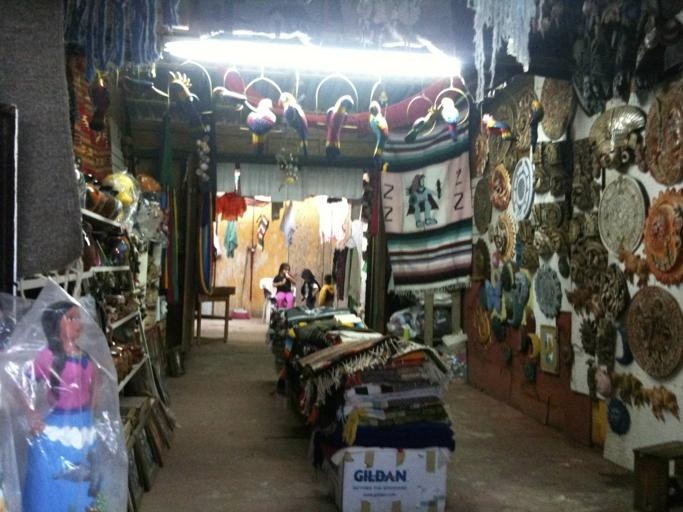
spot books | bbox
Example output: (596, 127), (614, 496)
(118, 391), (179, 511)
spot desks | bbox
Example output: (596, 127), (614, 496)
(192, 286), (236, 346)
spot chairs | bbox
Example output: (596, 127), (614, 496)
(257, 278), (297, 328)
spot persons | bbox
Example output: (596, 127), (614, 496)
(299, 269), (321, 308)
(5, 302), (103, 511)
(317, 274), (333, 307)
(271, 263), (297, 309)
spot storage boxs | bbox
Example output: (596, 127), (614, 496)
(316, 446), (448, 511)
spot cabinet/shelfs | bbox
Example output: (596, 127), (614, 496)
(81, 208), (156, 408)
